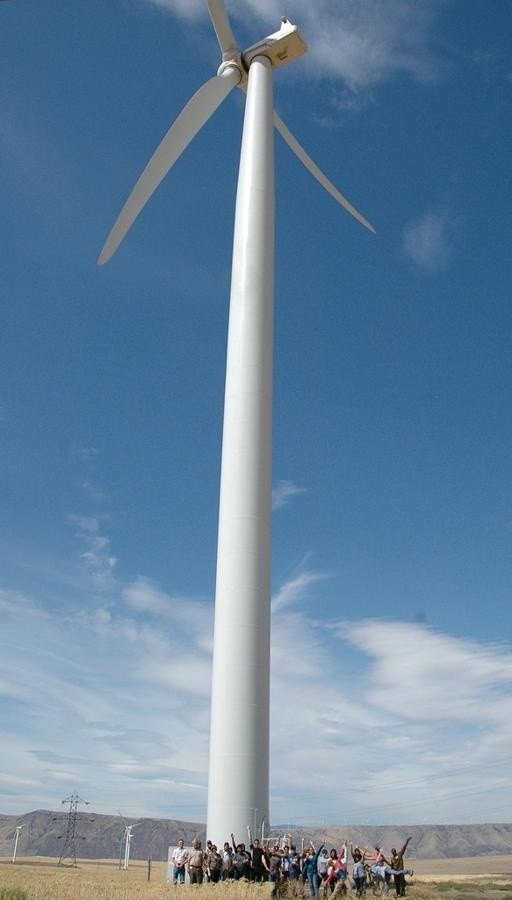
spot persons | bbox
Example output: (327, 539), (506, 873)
(168, 823), (416, 900)
